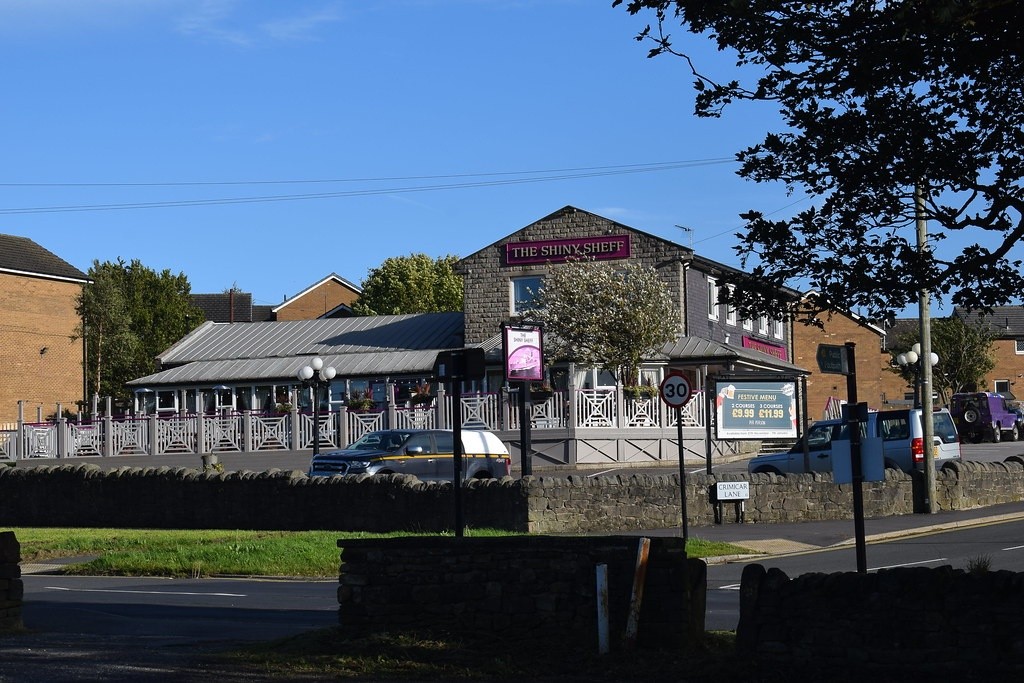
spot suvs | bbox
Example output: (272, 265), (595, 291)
(950, 390), (1024, 443)
(748, 409), (961, 475)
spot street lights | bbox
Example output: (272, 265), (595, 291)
(897, 343), (939, 408)
(298, 358), (337, 455)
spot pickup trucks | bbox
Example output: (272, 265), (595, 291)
(306, 431), (513, 480)
